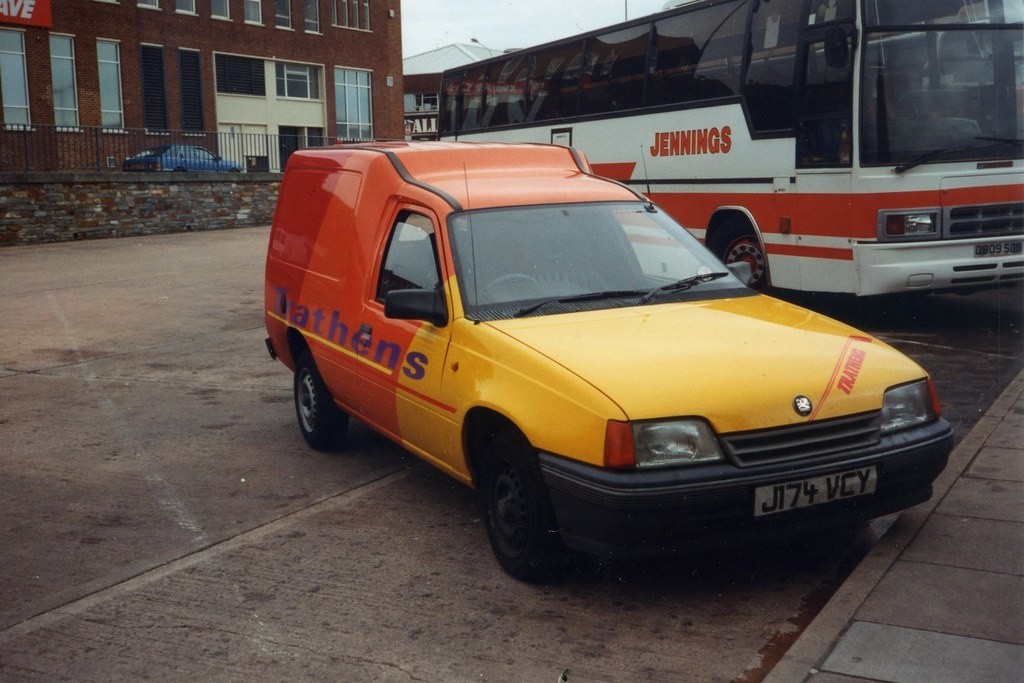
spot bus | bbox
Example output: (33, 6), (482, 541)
(435, 1), (1024, 308)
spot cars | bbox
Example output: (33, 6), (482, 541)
(121, 144), (244, 173)
(263, 142), (956, 587)
